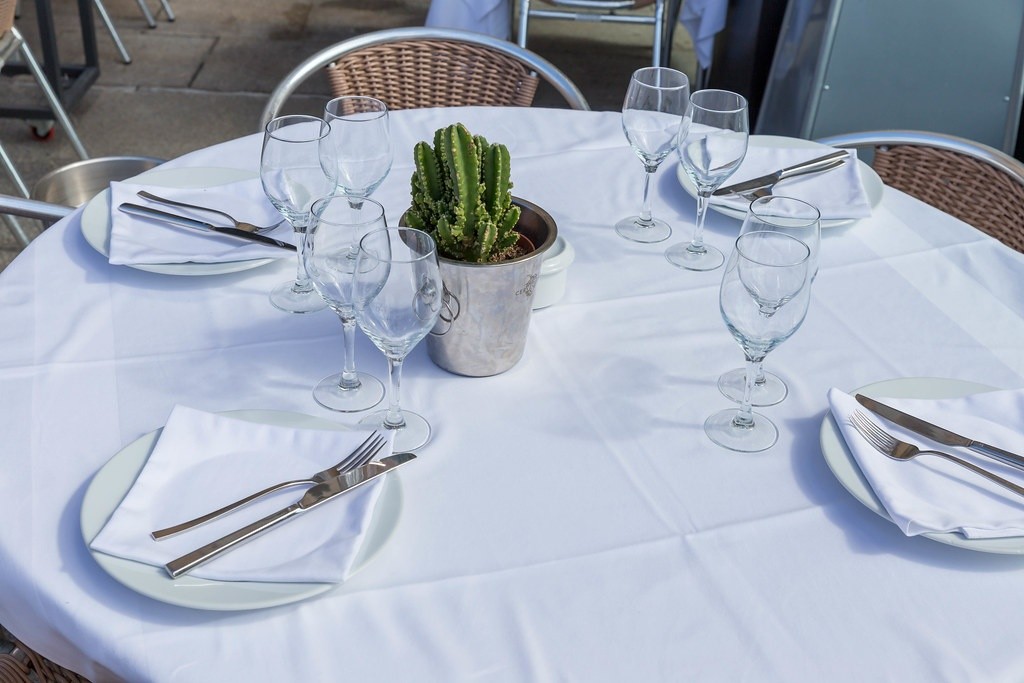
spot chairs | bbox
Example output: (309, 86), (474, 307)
(0, 0), (176, 248)
(260, 27), (590, 131)
(812, 129), (1024, 256)
(519, 0), (681, 112)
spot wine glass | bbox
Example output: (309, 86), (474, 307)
(304, 187), (387, 413)
(613, 67), (693, 242)
(349, 226), (442, 456)
(317, 95), (395, 274)
(703, 227), (814, 453)
(259, 117), (344, 313)
(664, 88), (750, 270)
(717, 197), (821, 405)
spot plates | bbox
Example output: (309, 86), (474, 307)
(79, 163), (298, 277)
(818, 374), (1024, 556)
(676, 134), (885, 229)
(82, 406), (405, 611)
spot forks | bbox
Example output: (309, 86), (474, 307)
(150, 423), (387, 545)
(728, 160), (847, 204)
(137, 188), (287, 235)
(846, 408), (1024, 505)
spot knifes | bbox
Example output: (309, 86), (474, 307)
(118, 202), (298, 255)
(852, 392), (1024, 473)
(164, 451), (419, 580)
(699, 150), (851, 198)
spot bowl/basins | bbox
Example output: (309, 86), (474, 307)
(27, 157), (168, 230)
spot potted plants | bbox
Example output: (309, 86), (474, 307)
(398, 123), (558, 380)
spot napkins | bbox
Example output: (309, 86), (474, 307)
(108, 175), (299, 266)
(827, 385), (1024, 541)
(91, 401), (399, 584)
(704, 133), (871, 221)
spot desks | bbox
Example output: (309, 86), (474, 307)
(0, 108), (1024, 683)
